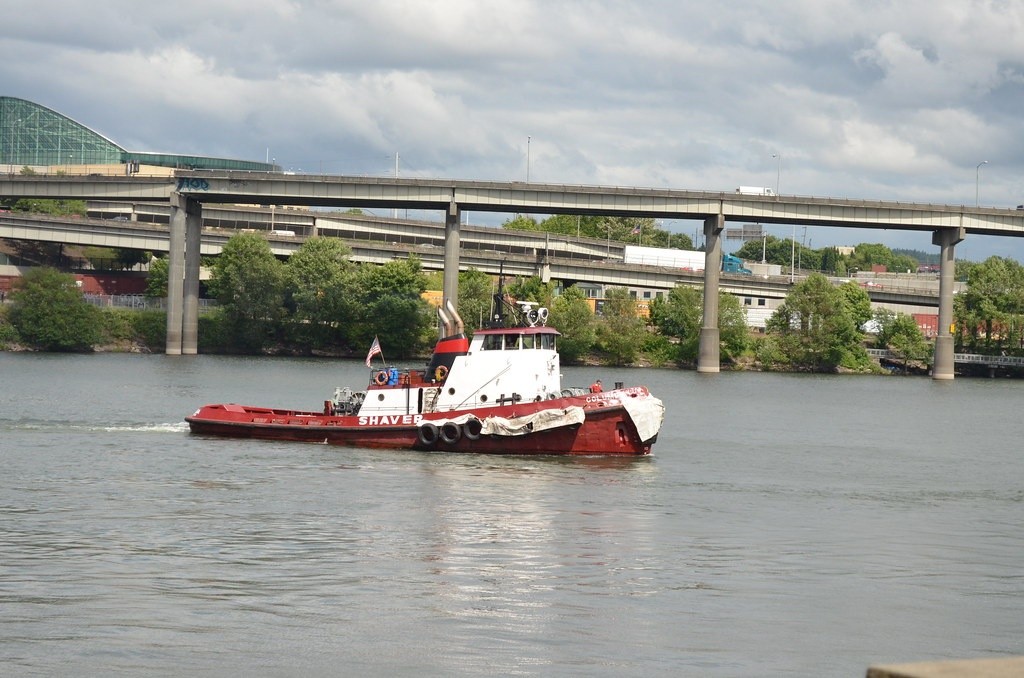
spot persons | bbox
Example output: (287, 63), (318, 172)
(589, 380), (603, 393)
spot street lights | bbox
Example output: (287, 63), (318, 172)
(976, 161), (988, 206)
(773, 154), (782, 195)
(527, 136), (531, 183)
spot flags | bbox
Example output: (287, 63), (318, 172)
(366, 334), (381, 368)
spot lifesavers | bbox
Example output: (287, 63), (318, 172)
(434, 364), (449, 380)
(374, 371), (390, 385)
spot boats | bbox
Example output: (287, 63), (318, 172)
(184, 257), (666, 455)
